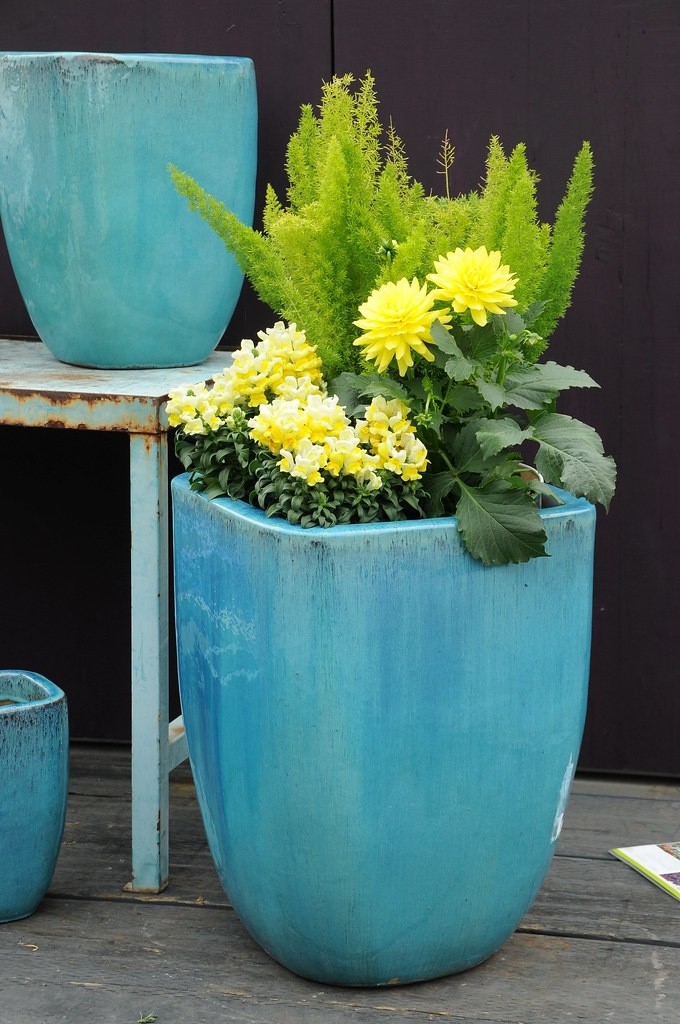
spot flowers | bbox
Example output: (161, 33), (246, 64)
(163, 65), (615, 568)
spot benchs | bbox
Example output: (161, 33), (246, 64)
(0, 339), (245, 890)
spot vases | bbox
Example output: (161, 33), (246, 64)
(0, 52), (257, 370)
(0, 670), (70, 924)
(172, 473), (598, 991)
